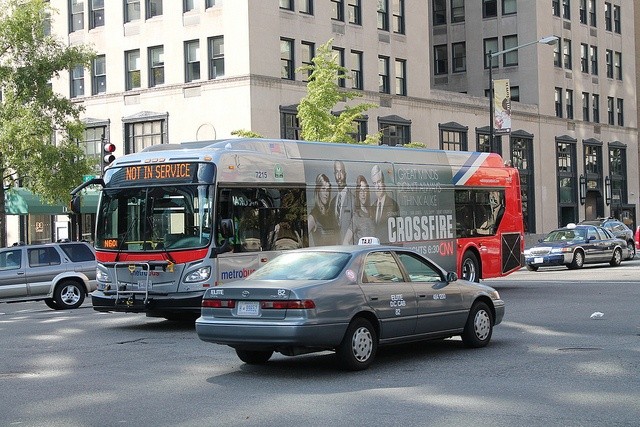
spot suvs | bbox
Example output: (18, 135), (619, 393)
(0, 241), (97, 311)
(576, 217), (636, 261)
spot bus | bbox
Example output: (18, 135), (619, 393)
(91, 137), (526, 318)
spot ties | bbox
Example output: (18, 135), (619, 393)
(337, 194), (342, 222)
(374, 199), (384, 227)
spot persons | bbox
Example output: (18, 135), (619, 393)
(369, 165), (400, 243)
(342, 176), (377, 245)
(306, 172), (338, 245)
(328, 159), (356, 245)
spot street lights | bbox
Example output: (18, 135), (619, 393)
(486, 34), (559, 152)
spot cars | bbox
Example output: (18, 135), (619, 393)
(195, 237), (506, 373)
(525, 222), (629, 272)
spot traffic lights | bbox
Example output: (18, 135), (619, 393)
(101, 142), (116, 175)
